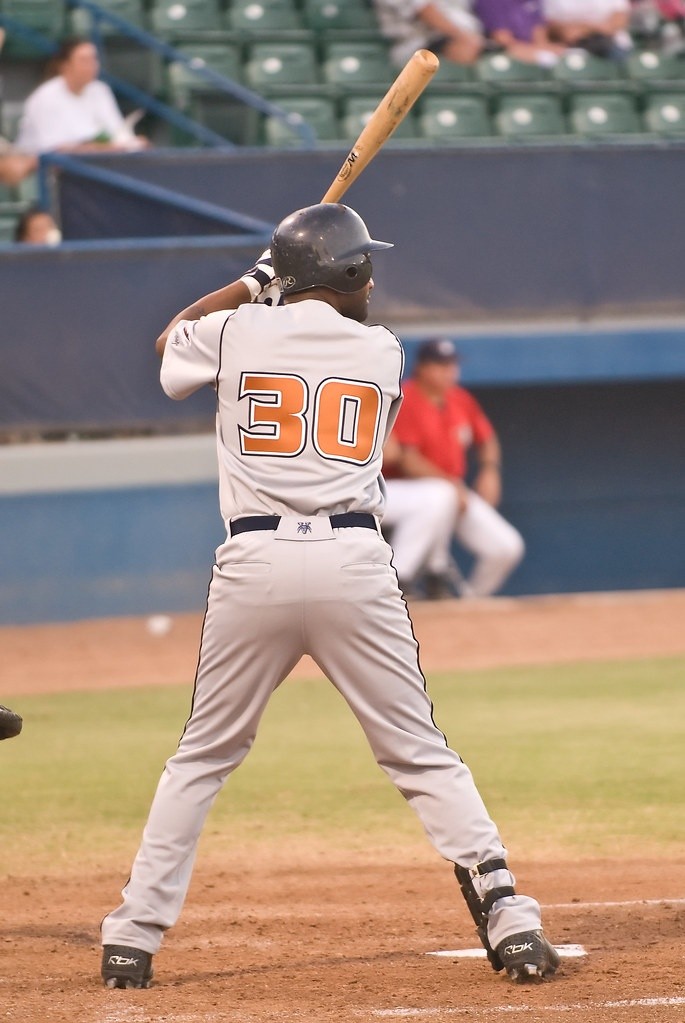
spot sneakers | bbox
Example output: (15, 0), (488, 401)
(101, 944), (154, 989)
(496, 929), (561, 983)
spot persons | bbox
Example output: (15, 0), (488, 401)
(13, 212), (62, 246)
(99, 204), (561, 988)
(373, 0), (685, 68)
(381, 336), (526, 601)
(15, 37), (145, 203)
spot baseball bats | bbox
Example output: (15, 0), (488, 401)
(320, 48), (440, 202)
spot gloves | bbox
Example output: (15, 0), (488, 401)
(240, 249), (276, 303)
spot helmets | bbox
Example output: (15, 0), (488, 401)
(271, 202), (394, 296)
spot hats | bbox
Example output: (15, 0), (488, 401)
(421, 340), (457, 364)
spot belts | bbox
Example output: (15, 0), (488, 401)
(230, 513), (377, 536)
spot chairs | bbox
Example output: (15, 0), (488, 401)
(0, 0), (685, 241)
